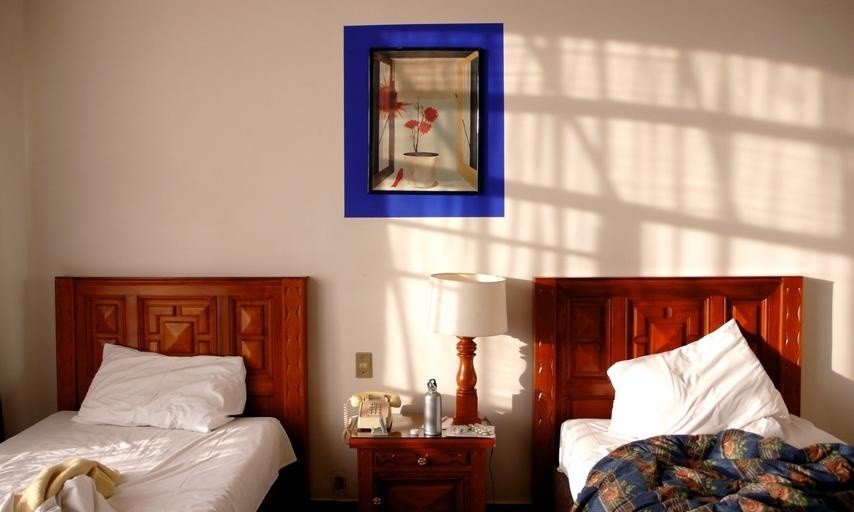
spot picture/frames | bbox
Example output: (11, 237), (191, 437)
(340, 22), (507, 220)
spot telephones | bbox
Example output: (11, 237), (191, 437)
(351, 391), (401, 432)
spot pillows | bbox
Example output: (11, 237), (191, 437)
(68, 341), (250, 434)
(603, 316), (792, 454)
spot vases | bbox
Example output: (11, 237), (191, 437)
(401, 154), (439, 190)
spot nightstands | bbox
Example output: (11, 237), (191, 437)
(347, 413), (496, 511)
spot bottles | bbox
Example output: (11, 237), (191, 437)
(424, 379), (441, 435)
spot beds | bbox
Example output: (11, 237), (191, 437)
(527, 273), (854, 511)
(0, 276), (313, 512)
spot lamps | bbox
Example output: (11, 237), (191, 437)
(428, 272), (510, 426)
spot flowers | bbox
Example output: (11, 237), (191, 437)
(401, 92), (441, 154)
